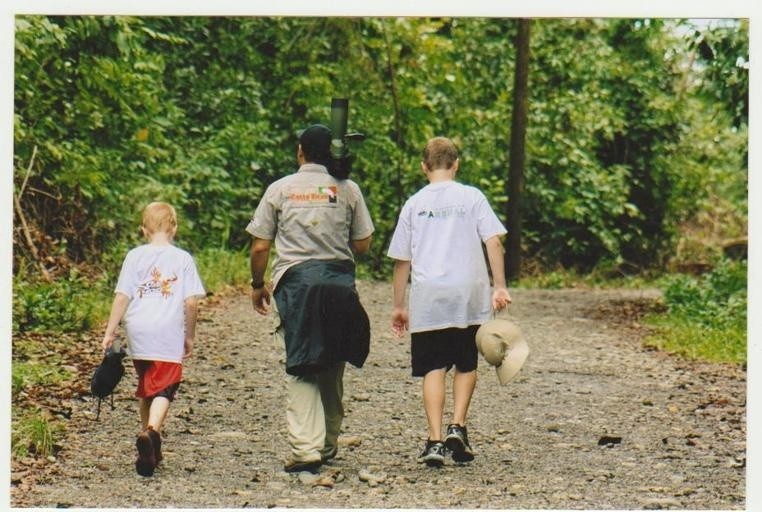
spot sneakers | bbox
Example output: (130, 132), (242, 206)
(135, 426), (162, 477)
(416, 437), (446, 464)
(444, 423), (474, 462)
(284, 457), (321, 474)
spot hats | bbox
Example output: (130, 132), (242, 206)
(295, 124), (333, 149)
(91, 351), (126, 399)
(475, 318), (530, 386)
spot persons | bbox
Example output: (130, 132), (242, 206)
(246, 125), (376, 471)
(101, 201), (206, 476)
(387, 137), (512, 464)
